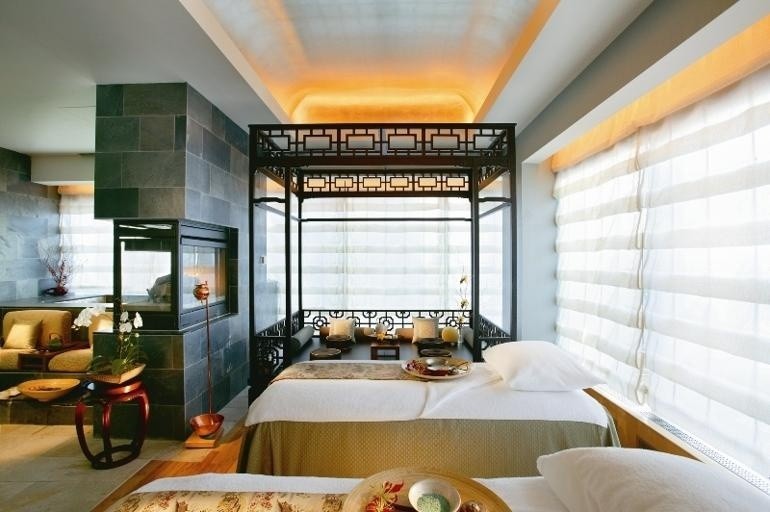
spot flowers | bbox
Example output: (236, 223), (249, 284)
(86, 311), (150, 374)
(456, 275), (471, 326)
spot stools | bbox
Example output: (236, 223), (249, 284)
(309, 347), (342, 359)
(75, 377), (149, 468)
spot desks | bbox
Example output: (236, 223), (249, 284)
(370, 341), (400, 360)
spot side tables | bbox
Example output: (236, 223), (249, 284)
(18, 341), (88, 372)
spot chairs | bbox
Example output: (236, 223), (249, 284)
(48, 312), (113, 373)
(0, 310), (72, 369)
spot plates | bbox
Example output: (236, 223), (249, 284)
(18, 378), (81, 401)
(427, 366), (454, 374)
(342, 465), (513, 512)
(426, 356), (448, 366)
(401, 357), (473, 379)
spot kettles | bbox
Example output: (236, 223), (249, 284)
(47, 332), (64, 351)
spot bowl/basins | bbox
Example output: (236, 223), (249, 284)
(408, 479), (461, 511)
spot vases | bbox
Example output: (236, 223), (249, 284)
(88, 362), (145, 384)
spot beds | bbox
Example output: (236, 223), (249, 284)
(247, 122), (517, 410)
(237, 359), (621, 479)
(102, 471), (566, 512)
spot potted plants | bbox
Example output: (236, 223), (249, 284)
(38, 236), (69, 295)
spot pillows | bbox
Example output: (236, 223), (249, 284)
(536, 447), (769, 512)
(411, 316), (439, 344)
(460, 327), (473, 347)
(290, 325), (315, 353)
(326, 320), (356, 343)
(482, 340), (608, 392)
(320, 326), (371, 336)
(396, 328), (443, 339)
(2, 318), (43, 349)
(441, 326), (458, 343)
